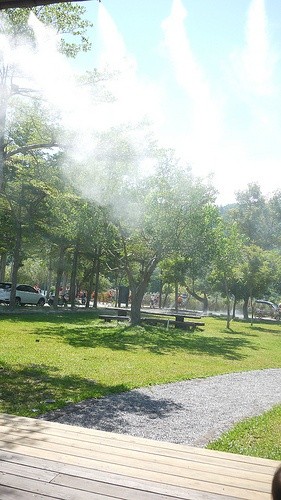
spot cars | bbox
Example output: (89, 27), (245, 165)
(0, 282), (46, 307)
(48, 293), (81, 305)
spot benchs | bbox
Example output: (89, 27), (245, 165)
(98, 315), (205, 330)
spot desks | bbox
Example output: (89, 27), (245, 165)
(104, 307), (201, 328)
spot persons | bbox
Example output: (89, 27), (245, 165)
(151, 295), (155, 304)
(107, 291), (113, 301)
(272, 467), (281, 500)
(82, 292), (86, 304)
(59, 288), (64, 295)
(33, 284), (39, 291)
(178, 295), (183, 308)
